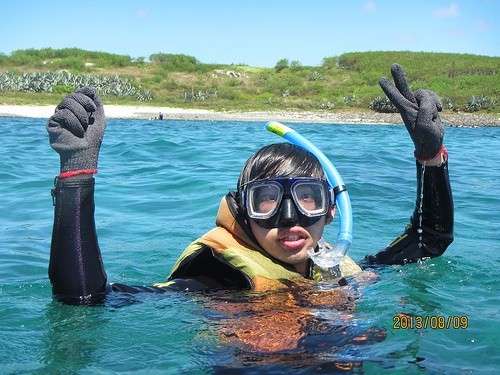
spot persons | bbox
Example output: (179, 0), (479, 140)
(47, 60), (456, 375)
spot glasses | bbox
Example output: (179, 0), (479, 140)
(239, 176), (336, 230)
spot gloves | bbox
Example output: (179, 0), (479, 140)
(44, 84), (105, 180)
(377, 64), (446, 164)
